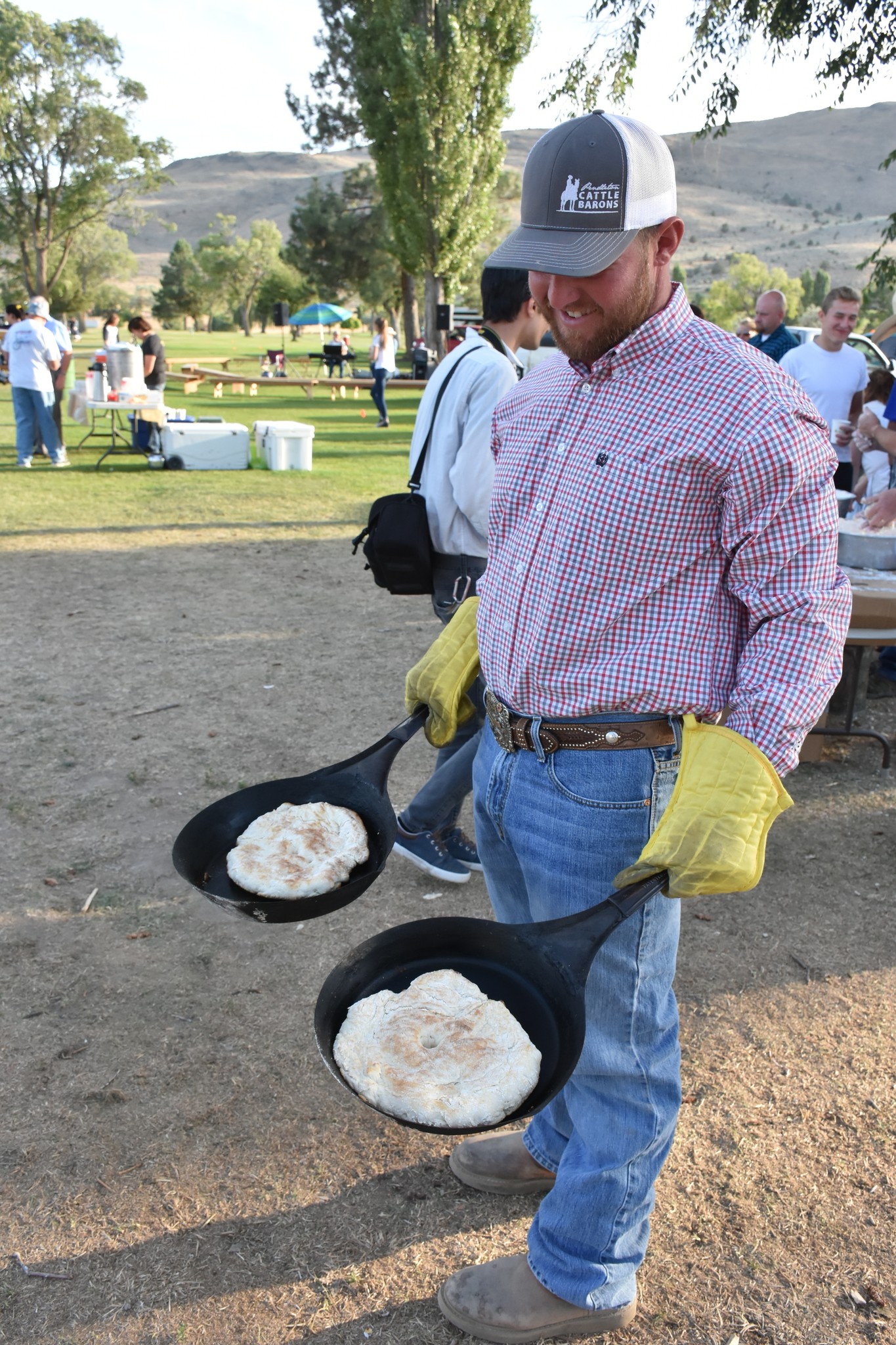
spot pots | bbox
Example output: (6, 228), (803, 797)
(313, 871), (672, 1136)
(170, 702), (429, 924)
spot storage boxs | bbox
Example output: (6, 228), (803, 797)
(253, 421), (315, 472)
(127, 413), (250, 470)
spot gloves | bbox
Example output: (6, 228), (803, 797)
(612, 705), (795, 901)
(405, 595), (484, 748)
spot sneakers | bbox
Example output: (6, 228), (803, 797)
(388, 811), (484, 884)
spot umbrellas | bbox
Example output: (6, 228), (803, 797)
(288, 301), (354, 376)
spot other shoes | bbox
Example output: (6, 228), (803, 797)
(376, 418), (390, 426)
(51, 460), (70, 468)
(17, 462), (32, 468)
(437, 1251), (638, 1344)
(449, 1127), (559, 1195)
(866, 659), (896, 697)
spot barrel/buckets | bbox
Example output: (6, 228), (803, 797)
(105, 347), (145, 391)
(126, 413), (196, 451)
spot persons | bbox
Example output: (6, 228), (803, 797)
(327, 331), (356, 378)
(370, 326), (400, 379)
(367, 316), (396, 428)
(747, 290), (801, 364)
(103, 313), (120, 350)
(128, 316), (167, 454)
(778, 286), (871, 493)
(853, 487), (896, 534)
(0, 302), (69, 468)
(67, 316), (82, 342)
(852, 378), (896, 489)
(4, 296), (73, 460)
(735, 317), (758, 343)
(391, 265), (552, 887)
(404, 112), (852, 1345)
(848, 369), (896, 516)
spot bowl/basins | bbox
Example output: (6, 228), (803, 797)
(148, 457), (165, 469)
(837, 531), (896, 571)
(835, 488), (857, 518)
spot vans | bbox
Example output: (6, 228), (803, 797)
(760, 327), (896, 376)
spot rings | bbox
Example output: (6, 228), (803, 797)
(881, 519), (887, 525)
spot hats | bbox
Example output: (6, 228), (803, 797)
(388, 327), (397, 335)
(24, 304), (50, 322)
(482, 108), (679, 278)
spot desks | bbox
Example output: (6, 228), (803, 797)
(67, 389), (165, 472)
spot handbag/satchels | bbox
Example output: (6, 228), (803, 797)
(351, 492), (437, 596)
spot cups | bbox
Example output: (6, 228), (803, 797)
(829, 418), (851, 444)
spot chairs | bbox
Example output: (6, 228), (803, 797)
(259, 350), (285, 372)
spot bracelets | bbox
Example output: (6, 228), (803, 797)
(871, 424), (881, 438)
(58, 371), (66, 376)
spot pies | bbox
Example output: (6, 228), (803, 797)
(334, 967), (542, 1128)
(226, 802), (369, 898)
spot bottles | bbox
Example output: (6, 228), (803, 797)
(85, 366), (93, 398)
(92, 362), (105, 402)
(95, 349), (107, 364)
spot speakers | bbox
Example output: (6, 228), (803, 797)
(436, 304), (454, 331)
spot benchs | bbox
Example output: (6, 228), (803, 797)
(163, 356), (429, 401)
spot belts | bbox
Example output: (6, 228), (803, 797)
(481, 689), (676, 755)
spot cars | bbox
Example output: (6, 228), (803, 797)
(412, 324), (524, 383)
